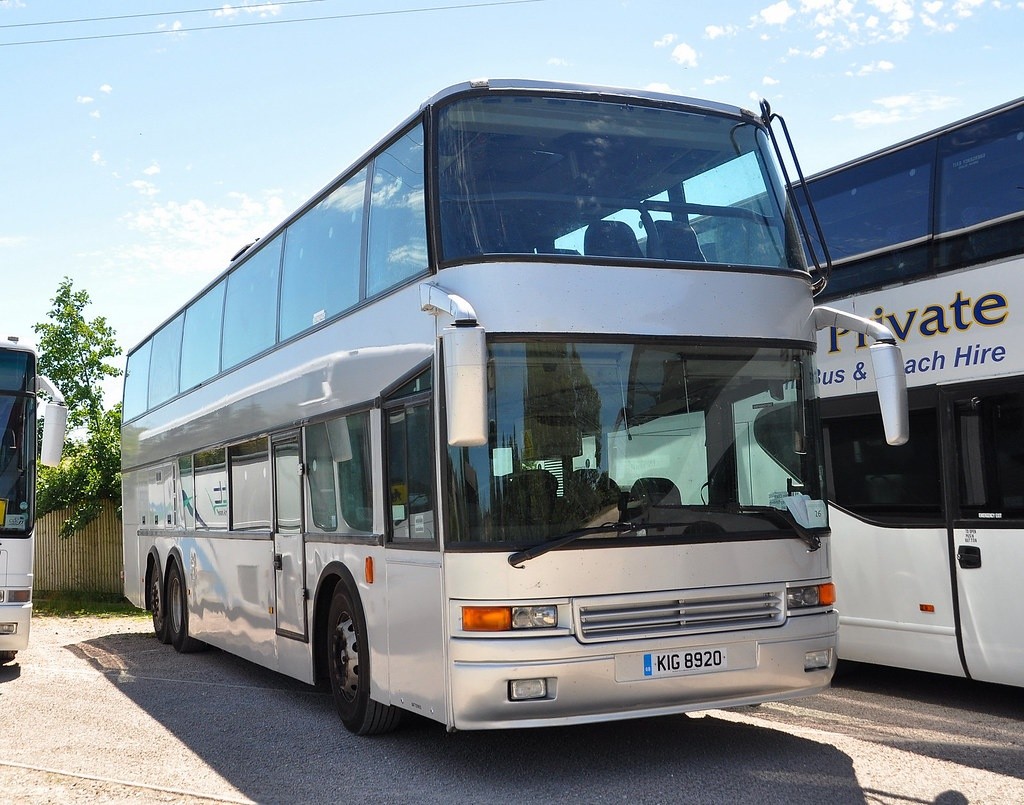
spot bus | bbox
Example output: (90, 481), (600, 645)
(121, 79), (910, 738)
(492, 94), (1024, 693)
(0, 336), (68, 666)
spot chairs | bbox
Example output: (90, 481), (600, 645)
(584, 220), (707, 263)
(484, 469), (682, 540)
